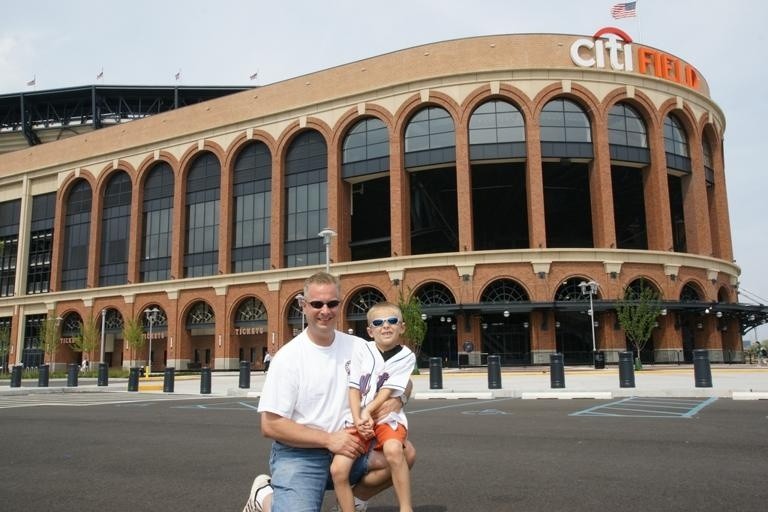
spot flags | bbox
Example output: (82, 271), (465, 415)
(249, 73), (257, 80)
(175, 72), (183, 80)
(612, 1), (637, 20)
(27, 78), (36, 86)
(95, 71), (103, 81)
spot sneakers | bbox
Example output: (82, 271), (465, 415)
(242, 474), (271, 512)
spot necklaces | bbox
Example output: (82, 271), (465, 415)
(308, 328), (335, 346)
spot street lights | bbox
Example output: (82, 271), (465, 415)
(144, 307), (159, 373)
(296, 293), (306, 332)
(101, 308), (106, 363)
(578, 280), (600, 365)
(319, 227), (338, 274)
(749, 315), (759, 343)
(47, 313), (62, 373)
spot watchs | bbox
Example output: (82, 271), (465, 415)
(398, 393), (408, 407)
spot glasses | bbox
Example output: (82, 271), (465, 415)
(369, 317), (399, 328)
(310, 301), (339, 308)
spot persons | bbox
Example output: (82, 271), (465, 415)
(241, 270), (412, 512)
(262, 352), (271, 373)
(754, 341), (768, 368)
(329, 301), (412, 512)
(81, 357), (87, 374)
(85, 358), (89, 373)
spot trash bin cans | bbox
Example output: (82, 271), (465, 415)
(595, 351), (604, 369)
